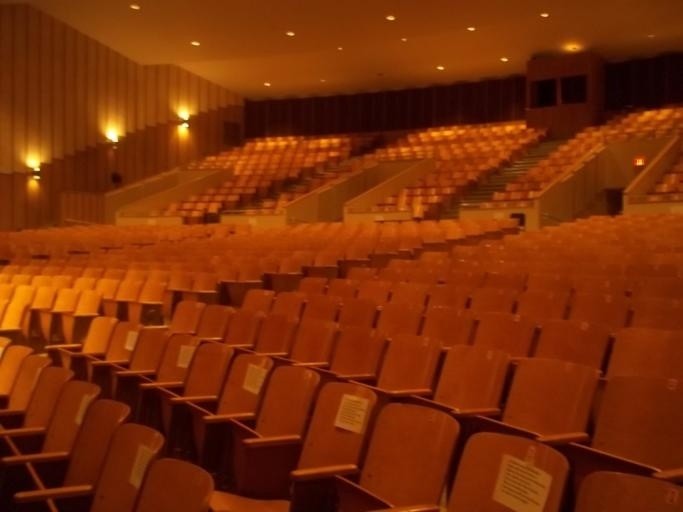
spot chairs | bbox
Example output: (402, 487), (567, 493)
(0, 102), (681, 512)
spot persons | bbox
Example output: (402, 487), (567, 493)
(110, 173), (123, 189)
